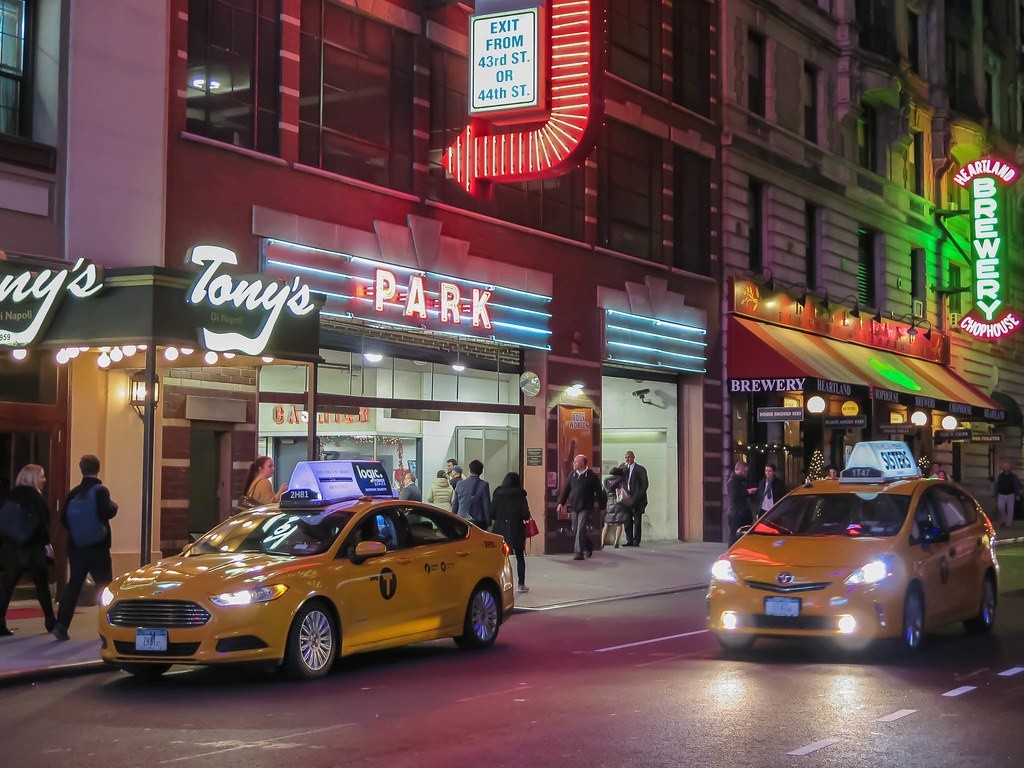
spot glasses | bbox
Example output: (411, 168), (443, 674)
(403, 477), (410, 480)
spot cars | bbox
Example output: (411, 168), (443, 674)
(96, 458), (516, 683)
(706, 441), (1000, 660)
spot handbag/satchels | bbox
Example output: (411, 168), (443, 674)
(0, 501), (41, 542)
(615, 482), (633, 507)
(464, 514), (483, 528)
(522, 516), (539, 538)
(234, 478), (266, 515)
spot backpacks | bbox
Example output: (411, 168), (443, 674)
(67, 484), (108, 546)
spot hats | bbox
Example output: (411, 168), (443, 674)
(452, 466), (462, 473)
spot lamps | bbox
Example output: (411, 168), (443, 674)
(747, 267), (774, 292)
(891, 313), (918, 335)
(933, 416), (958, 447)
(806, 395), (826, 416)
(841, 295), (860, 318)
(917, 320), (932, 341)
(788, 283), (808, 309)
(451, 363), (468, 371)
(809, 286), (829, 311)
(363, 353), (382, 362)
(911, 411), (927, 441)
(860, 303), (882, 325)
(837, 401), (859, 434)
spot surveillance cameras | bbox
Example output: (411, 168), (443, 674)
(632, 389), (650, 397)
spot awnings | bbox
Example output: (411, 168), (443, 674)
(726, 315), (1006, 422)
(990, 392), (1023, 426)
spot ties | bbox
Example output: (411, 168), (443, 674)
(625, 466), (631, 490)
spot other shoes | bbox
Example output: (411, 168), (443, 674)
(53, 624), (70, 640)
(586, 542), (594, 557)
(574, 555), (585, 560)
(635, 541), (640, 546)
(614, 542), (619, 548)
(517, 584), (529, 592)
(622, 541), (634, 546)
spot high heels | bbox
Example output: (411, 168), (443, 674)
(0, 627), (14, 636)
(46, 622), (56, 633)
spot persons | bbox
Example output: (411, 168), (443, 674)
(828, 465), (841, 478)
(488, 472), (530, 592)
(622, 450), (648, 547)
(447, 459), (466, 480)
(452, 459), (492, 531)
(0, 464), (57, 636)
(601, 462), (625, 548)
(400, 473), (421, 523)
(930, 462), (951, 480)
(428, 470), (455, 513)
(277, 515), (369, 552)
(52, 454), (119, 640)
(993, 463), (1023, 527)
(727, 461), (757, 549)
(244, 455), (288, 505)
(755, 463), (785, 519)
(450, 466), (463, 490)
(556, 454), (606, 560)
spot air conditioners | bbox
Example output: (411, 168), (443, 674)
(912, 299), (923, 319)
(950, 313), (963, 328)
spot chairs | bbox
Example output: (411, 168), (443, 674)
(357, 516), (382, 542)
(812, 497), (848, 529)
(410, 521), (440, 540)
